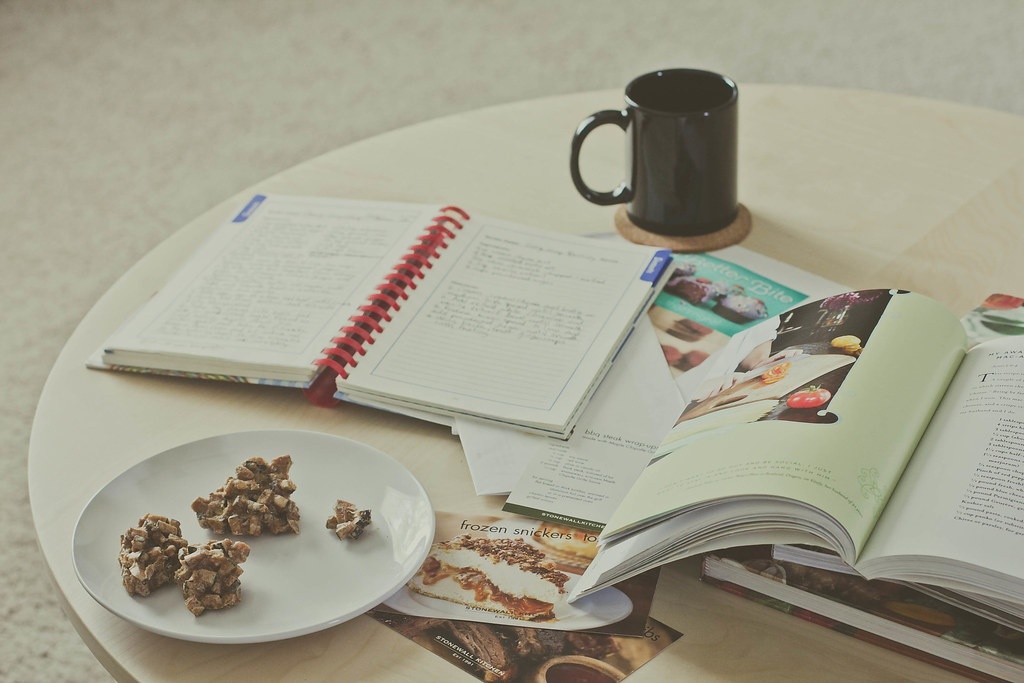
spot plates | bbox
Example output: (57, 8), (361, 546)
(72, 431), (435, 642)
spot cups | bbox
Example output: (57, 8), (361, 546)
(571, 68), (739, 237)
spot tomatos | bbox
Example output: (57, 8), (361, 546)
(788, 385), (832, 409)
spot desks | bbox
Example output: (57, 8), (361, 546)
(26, 84), (1024, 683)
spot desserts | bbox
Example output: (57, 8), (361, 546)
(404, 533), (570, 621)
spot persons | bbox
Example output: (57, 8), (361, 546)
(692, 314), (803, 402)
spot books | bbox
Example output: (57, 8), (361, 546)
(85, 191), (680, 440)
(567, 290), (1024, 683)
